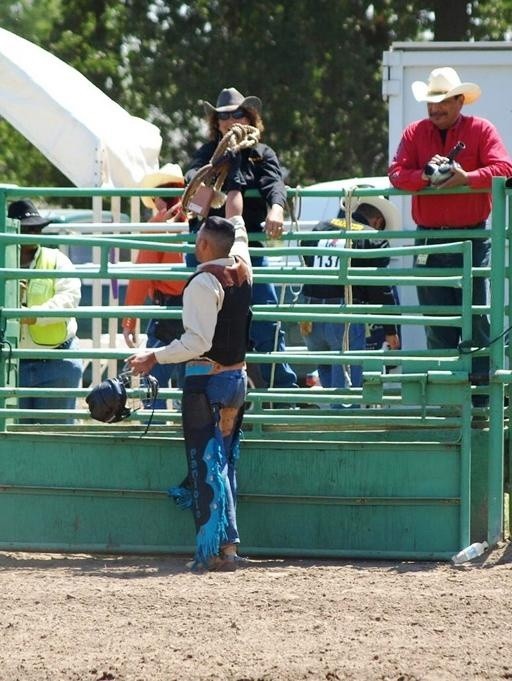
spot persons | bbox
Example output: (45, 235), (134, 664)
(119, 163), (184, 427)
(187, 84), (299, 412)
(125, 139), (254, 569)
(7, 197), (87, 426)
(301, 188), (401, 409)
(387, 66), (512, 418)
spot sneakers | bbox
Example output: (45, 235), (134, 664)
(185, 545), (237, 572)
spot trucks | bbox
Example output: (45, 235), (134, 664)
(264, 37), (512, 414)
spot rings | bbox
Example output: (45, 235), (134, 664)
(277, 227), (282, 230)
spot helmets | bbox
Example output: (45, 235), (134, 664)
(86, 372), (158, 424)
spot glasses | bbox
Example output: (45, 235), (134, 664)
(217, 109), (247, 120)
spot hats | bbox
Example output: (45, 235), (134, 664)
(139, 163), (185, 208)
(412, 68), (481, 104)
(7, 200), (52, 229)
(203, 86), (262, 123)
(349, 196), (402, 230)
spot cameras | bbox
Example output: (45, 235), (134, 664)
(421, 141), (465, 184)
